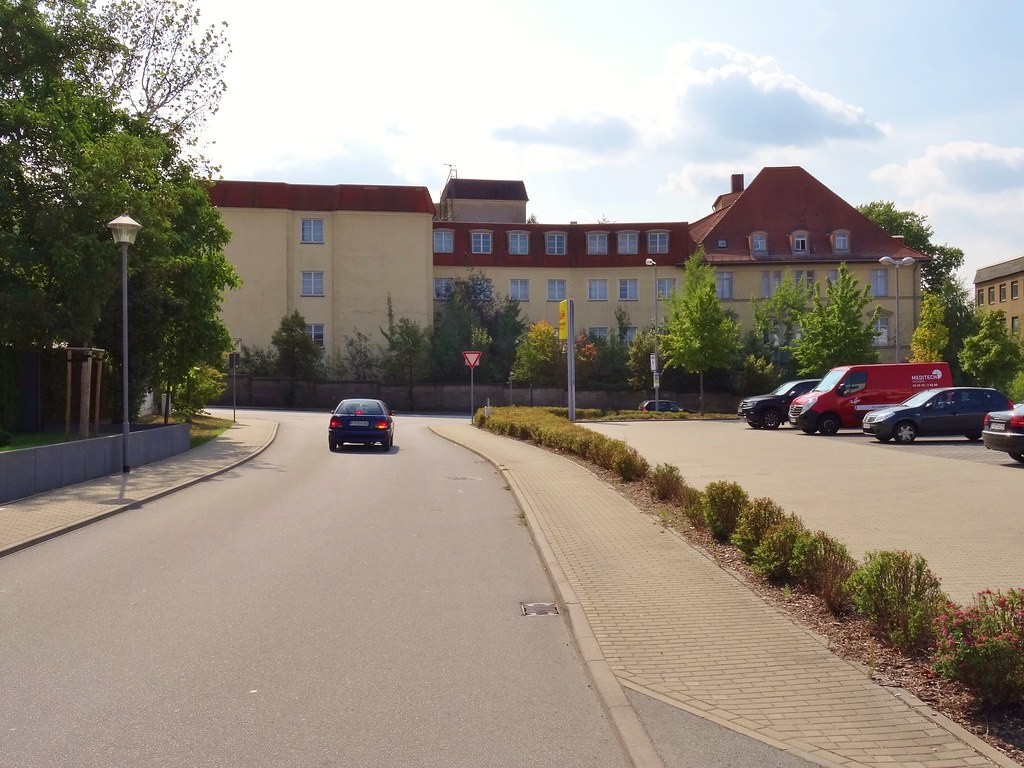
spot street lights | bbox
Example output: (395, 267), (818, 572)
(646, 257), (660, 412)
(105, 214), (142, 474)
(879, 254), (915, 362)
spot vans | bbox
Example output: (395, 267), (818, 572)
(788, 362), (953, 436)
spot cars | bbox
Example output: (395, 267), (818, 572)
(328, 398), (398, 452)
(982, 405), (1024, 462)
(861, 387), (1014, 444)
(639, 400), (698, 414)
(737, 379), (821, 428)
